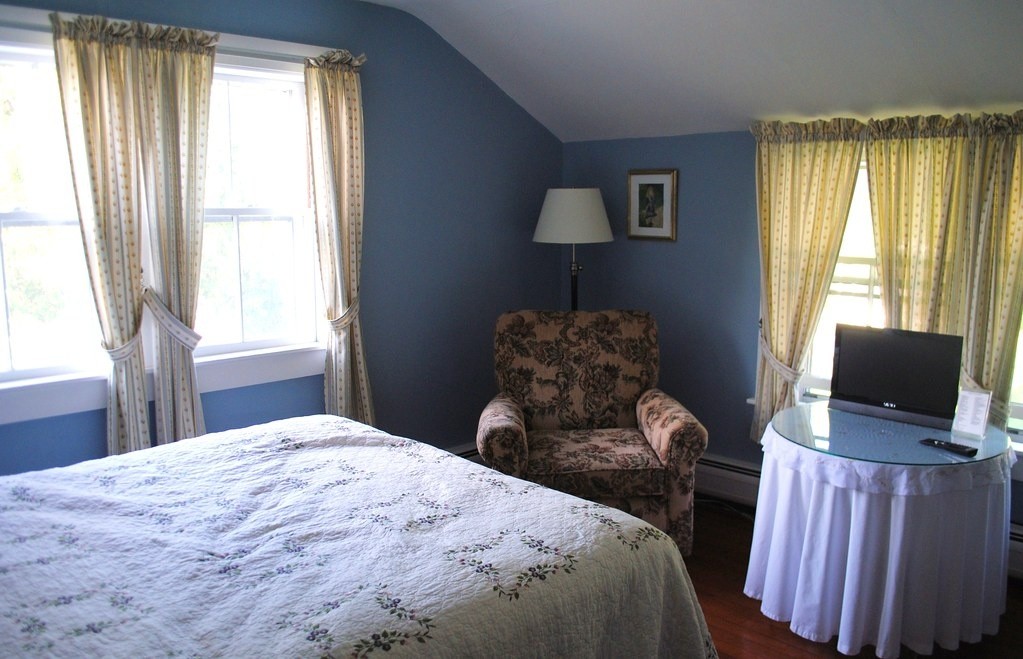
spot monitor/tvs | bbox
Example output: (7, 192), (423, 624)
(828, 324), (963, 431)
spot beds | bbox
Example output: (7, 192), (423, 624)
(0, 414), (718, 659)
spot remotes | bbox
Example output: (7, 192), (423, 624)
(918, 438), (978, 458)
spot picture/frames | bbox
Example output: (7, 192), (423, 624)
(626, 168), (677, 240)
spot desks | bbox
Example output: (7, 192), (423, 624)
(742, 400), (1018, 659)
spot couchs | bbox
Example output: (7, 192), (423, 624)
(476, 310), (708, 555)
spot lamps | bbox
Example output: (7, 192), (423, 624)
(532, 189), (614, 311)
